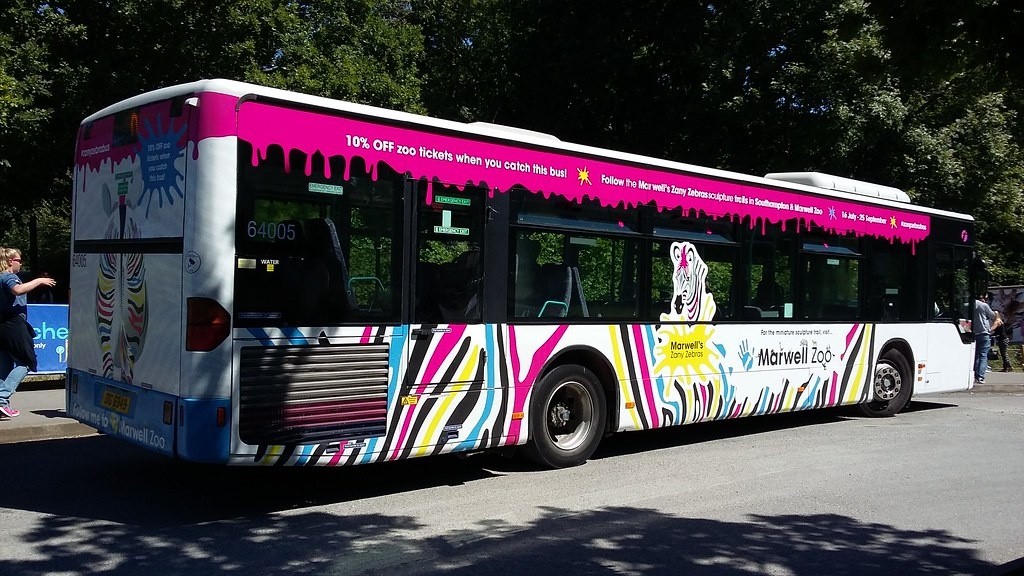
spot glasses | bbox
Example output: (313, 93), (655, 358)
(6, 258), (22, 264)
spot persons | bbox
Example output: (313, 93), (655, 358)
(264, 220), (328, 326)
(987, 310), (1013, 372)
(519, 240), (549, 297)
(966, 292), (997, 384)
(751, 260), (785, 310)
(0, 246), (57, 418)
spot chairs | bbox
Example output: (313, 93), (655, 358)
(276, 215), (786, 320)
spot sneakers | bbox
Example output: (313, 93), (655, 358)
(0, 409), (21, 417)
(0, 406), (13, 417)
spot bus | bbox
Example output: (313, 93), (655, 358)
(70, 78), (987, 466)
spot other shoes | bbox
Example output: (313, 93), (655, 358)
(985, 366), (993, 373)
(976, 376), (986, 383)
(998, 368), (1008, 372)
(1008, 366), (1014, 372)
